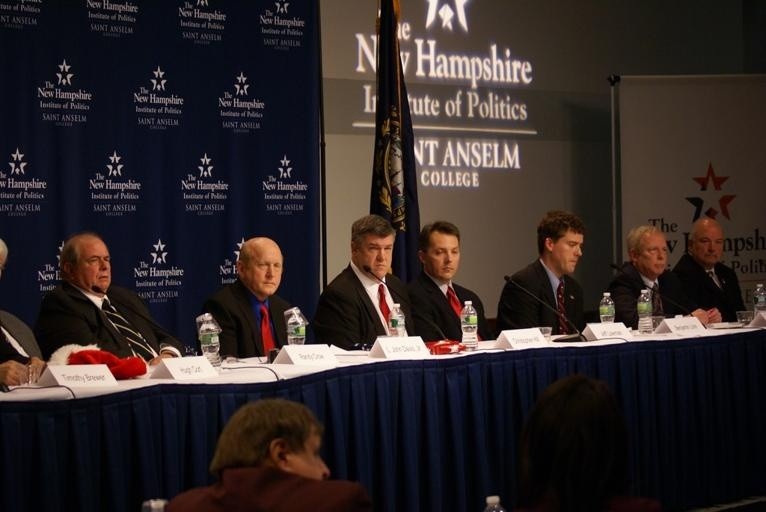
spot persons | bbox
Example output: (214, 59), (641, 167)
(32, 229), (188, 368)
(160, 396), (380, 512)
(401, 219), (493, 343)
(663, 215), (750, 321)
(503, 372), (671, 511)
(601, 222), (725, 330)
(0, 236), (48, 391)
(196, 234), (319, 360)
(493, 206), (592, 338)
(309, 212), (417, 352)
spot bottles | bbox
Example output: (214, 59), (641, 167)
(600, 292), (616, 322)
(387, 302), (406, 336)
(752, 284), (766, 323)
(198, 312), (221, 371)
(459, 299), (478, 350)
(482, 493), (506, 511)
(285, 309), (305, 344)
(636, 288), (654, 333)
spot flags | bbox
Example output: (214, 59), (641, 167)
(367, 1), (422, 282)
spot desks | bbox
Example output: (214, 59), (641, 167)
(0, 318), (765, 511)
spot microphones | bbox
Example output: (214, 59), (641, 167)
(363, 264), (446, 339)
(92, 285), (196, 356)
(504, 274), (587, 343)
(608, 262), (707, 330)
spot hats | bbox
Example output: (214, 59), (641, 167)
(47, 344), (146, 379)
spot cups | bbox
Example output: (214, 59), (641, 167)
(540, 326), (552, 343)
(652, 315), (665, 331)
(735, 310), (754, 326)
(283, 307), (310, 331)
(195, 312), (223, 340)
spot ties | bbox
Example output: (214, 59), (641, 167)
(652, 282), (664, 316)
(708, 272), (723, 289)
(260, 305), (275, 356)
(557, 280), (569, 335)
(102, 298), (154, 361)
(378, 284), (392, 328)
(447, 286), (480, 341)
